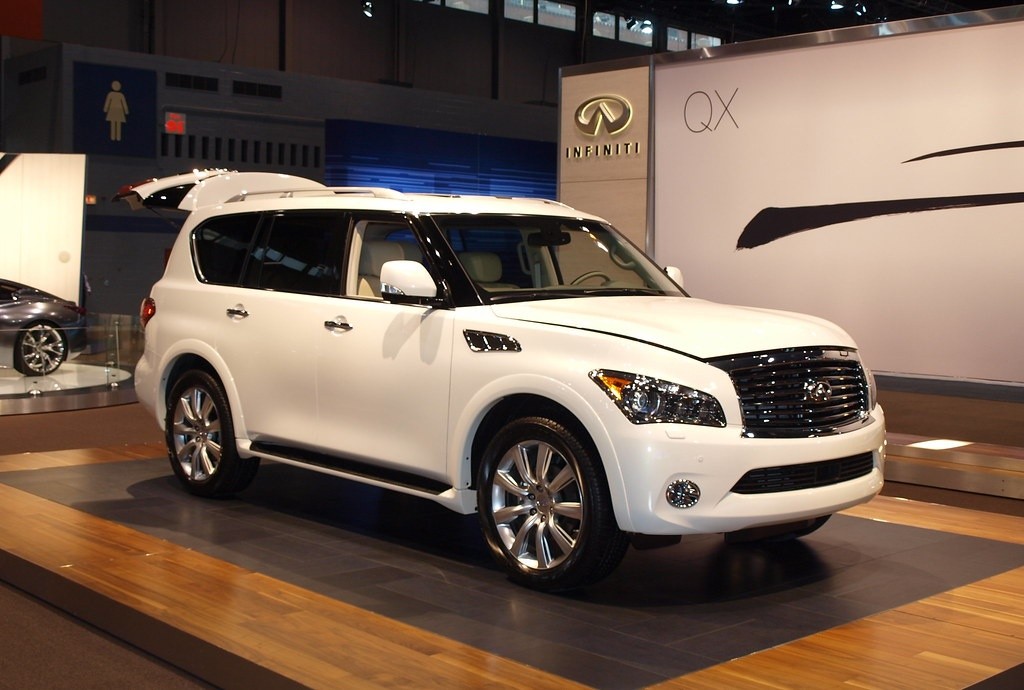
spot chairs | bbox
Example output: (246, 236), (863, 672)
(458, 252), (519, 292)
(358, 240), (423, 298)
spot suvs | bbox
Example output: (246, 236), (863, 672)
(111, 170), (887, 593)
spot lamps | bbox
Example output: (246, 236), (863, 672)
(623, 16), (638, 31)
(640, 21), (653, 33)
(362, 0), (373, 18)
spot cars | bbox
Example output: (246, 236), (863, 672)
(0, 278), (86, 376)
